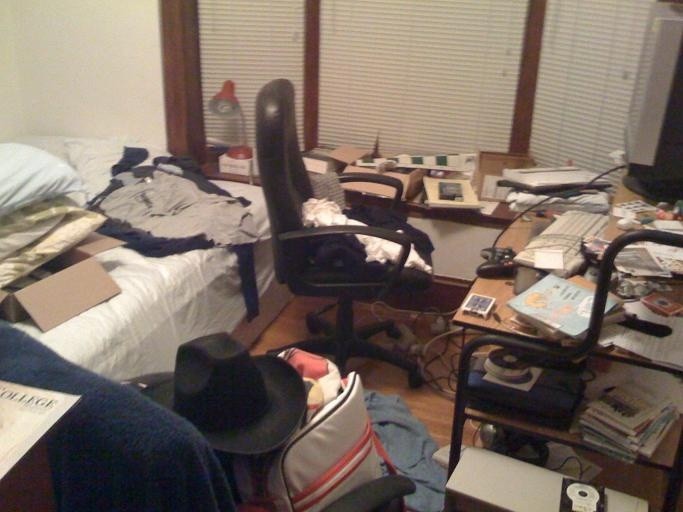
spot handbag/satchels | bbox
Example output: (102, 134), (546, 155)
(231, 347), (415, 512)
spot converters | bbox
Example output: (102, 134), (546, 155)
(386, 325), (402, 339)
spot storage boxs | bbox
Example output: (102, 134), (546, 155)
(331, 143), (428, 203)
(10, 177), (272, 385)
(475, 151), (536, 202)
(218, 149), (260, 177)
(302, 146), (339, 177)
(0, 232), (124, 333)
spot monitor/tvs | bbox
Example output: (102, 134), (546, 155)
(619, 8), (683, 206)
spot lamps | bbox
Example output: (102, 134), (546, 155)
(209, 78), (253, 159)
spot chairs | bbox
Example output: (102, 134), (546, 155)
(253, 78), (434, 389)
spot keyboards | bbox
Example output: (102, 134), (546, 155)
(511, 208), (612, 280)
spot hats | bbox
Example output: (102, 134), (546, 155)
(165, 333), (307, 455)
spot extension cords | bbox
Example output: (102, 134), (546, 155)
(393, 322), (418, 349)
(430, 320), (484, 335)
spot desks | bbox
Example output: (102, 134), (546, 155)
(445, 201), (682, 512)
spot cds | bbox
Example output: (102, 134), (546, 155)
(484, 348), (530, 381)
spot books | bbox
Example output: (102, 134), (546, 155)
(299, 144), (343, 174)
(576, 379), (679, 469)
(600, 196), (682, 279)
(505, 273), (681, 372)
(420, 174), (482, 211)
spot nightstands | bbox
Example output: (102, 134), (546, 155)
(0, 381), (86, 512)
